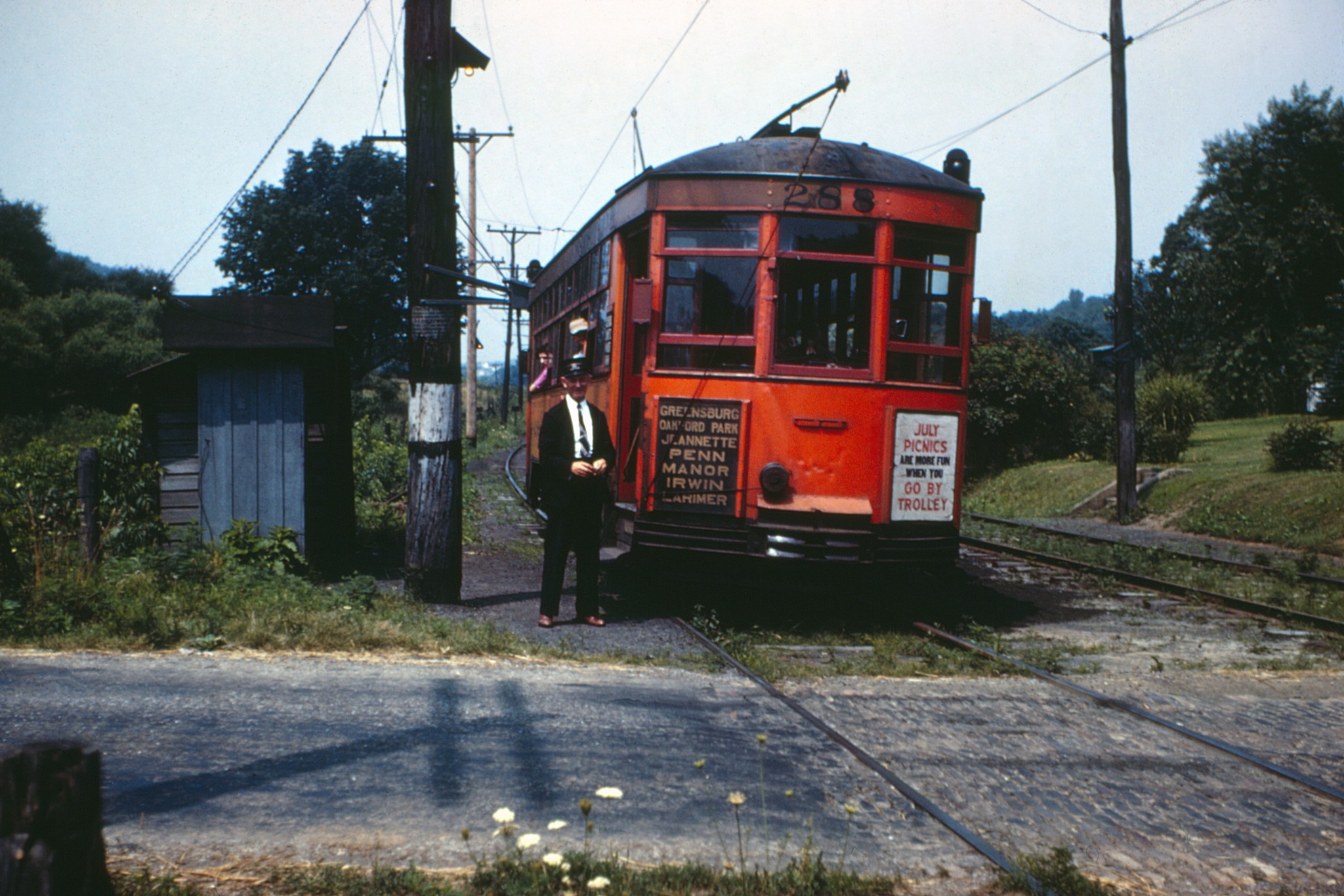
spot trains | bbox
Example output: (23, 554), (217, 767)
(515, 69), (990, 595)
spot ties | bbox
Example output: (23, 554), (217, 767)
(577, 402), (590, 459)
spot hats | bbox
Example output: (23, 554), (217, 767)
(559, 357), (589, 378)
(569, 317), (588, 335)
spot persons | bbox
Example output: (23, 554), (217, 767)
(567, 317), (589, 376)
(538, 358), (617, 628)
(529, 343), (553, 395)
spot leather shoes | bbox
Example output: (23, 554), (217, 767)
(584, 615), (605, 626)
(539, 614), (552, 627)
